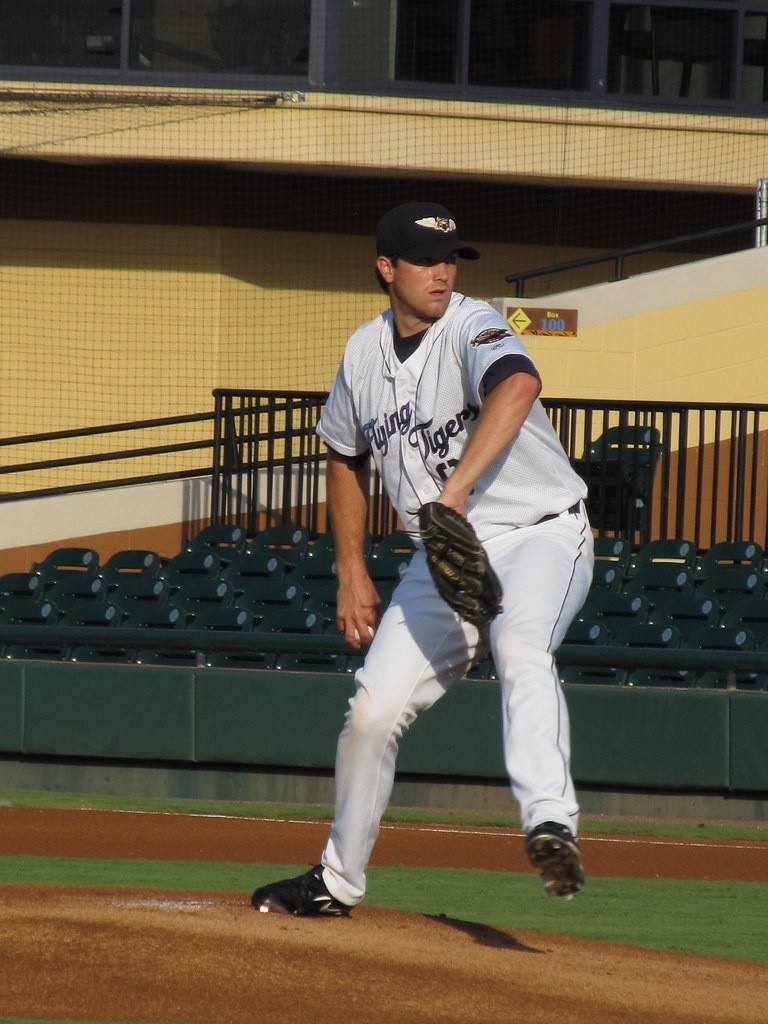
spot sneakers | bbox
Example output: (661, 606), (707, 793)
(524, 824), (586, 900)
(252, 864), (350, 914)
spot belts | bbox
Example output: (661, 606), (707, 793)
(535, 500), (580, 526)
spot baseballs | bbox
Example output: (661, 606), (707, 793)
(355, 625), (374, 643)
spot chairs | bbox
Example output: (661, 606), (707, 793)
(0, 523), (768, 693)
(566, 425), (662, 547)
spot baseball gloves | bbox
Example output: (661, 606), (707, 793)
(408, 501), (504, 626)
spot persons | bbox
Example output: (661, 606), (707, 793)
(251, 197), (593, 918)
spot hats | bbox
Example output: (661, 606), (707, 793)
(376, 202), (481, 263)
